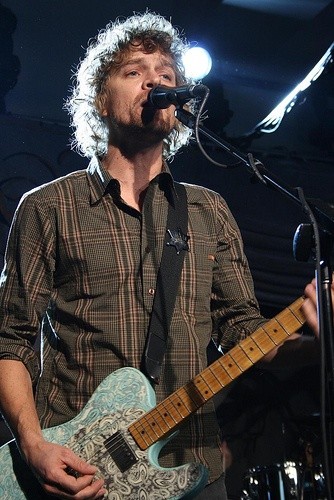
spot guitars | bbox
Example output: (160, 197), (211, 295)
(0, 289), (315, 500)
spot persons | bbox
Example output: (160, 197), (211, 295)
(0, 15), (334, 500)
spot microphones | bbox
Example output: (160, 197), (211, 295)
(147, 83), (208, 108)
